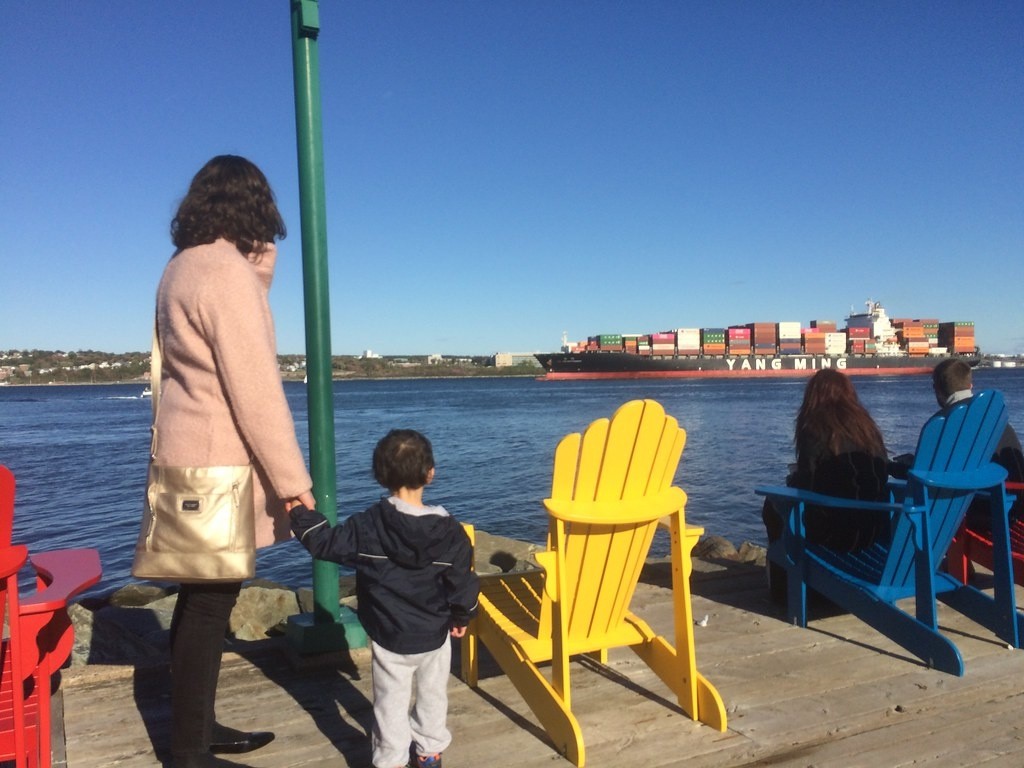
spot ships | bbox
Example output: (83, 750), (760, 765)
(532, 300), (986, 380)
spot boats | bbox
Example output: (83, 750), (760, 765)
(142, 387), (153, 397)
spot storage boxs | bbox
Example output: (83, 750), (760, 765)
(574, 319), (879, 355)
(891, 318), (975, 356)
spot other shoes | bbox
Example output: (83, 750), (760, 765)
(409, 743), (441, 767)
(769, 583), (789, 605)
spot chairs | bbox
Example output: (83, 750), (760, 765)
(754, 386), (1024, 678)
(946, 482), (1024, 586)
(0, 464), (104, 768)
(462, 399), (728, 768)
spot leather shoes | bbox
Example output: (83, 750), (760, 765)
(209, 730), (275, 755)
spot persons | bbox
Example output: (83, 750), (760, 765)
(890, 359), (1024, 590)
(286, 429), (481, 768)
(762, 369), (890, 606)
(131, 153), (316, 768)
